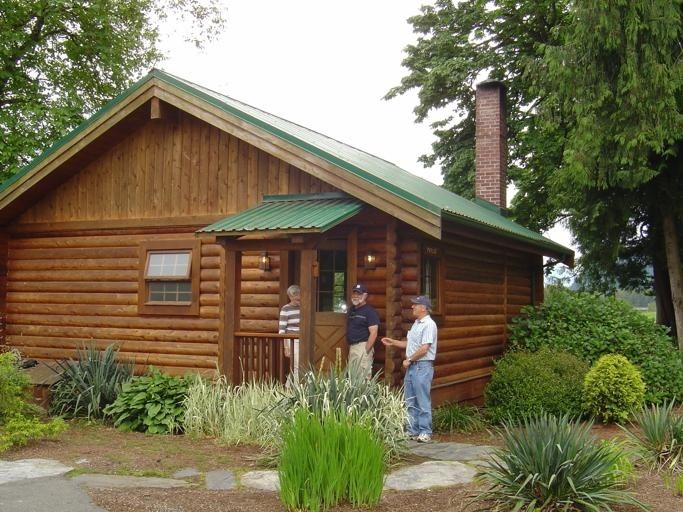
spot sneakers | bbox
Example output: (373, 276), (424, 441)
(416, 433), (430, 442)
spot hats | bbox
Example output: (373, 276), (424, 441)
(352, 283), (367, 293)
(409, 295), (431, 308)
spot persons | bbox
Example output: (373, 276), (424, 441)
(380, 296), (438, 445)
(344, 283), (379, 387)
(277, 285), (300, 389)
